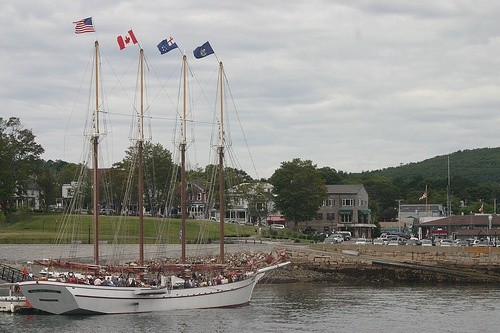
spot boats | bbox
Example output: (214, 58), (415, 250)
(0, 296), (33, 313)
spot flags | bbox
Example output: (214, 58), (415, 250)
(73, 17), (95, 34)
(117, 29), (138, 51)
(193, 41), (215, 59)
(420, 189), (427, 200)
(157, 36), (178, 55)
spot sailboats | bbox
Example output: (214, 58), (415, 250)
(3, 40), (291, 315)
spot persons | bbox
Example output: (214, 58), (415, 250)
(13, 267), (250, 291)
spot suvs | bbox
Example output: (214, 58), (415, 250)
(319, 230), (352, 243)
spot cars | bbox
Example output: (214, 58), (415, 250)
(74, 209), (89, 214)
(356, 233), (432, 247)
(227, 220), (239, 224)
(98, 209), (116, 215)
(434, 238), (500, 247)
(269, 224), (284, 230)
(144, 212), (164, 217)
